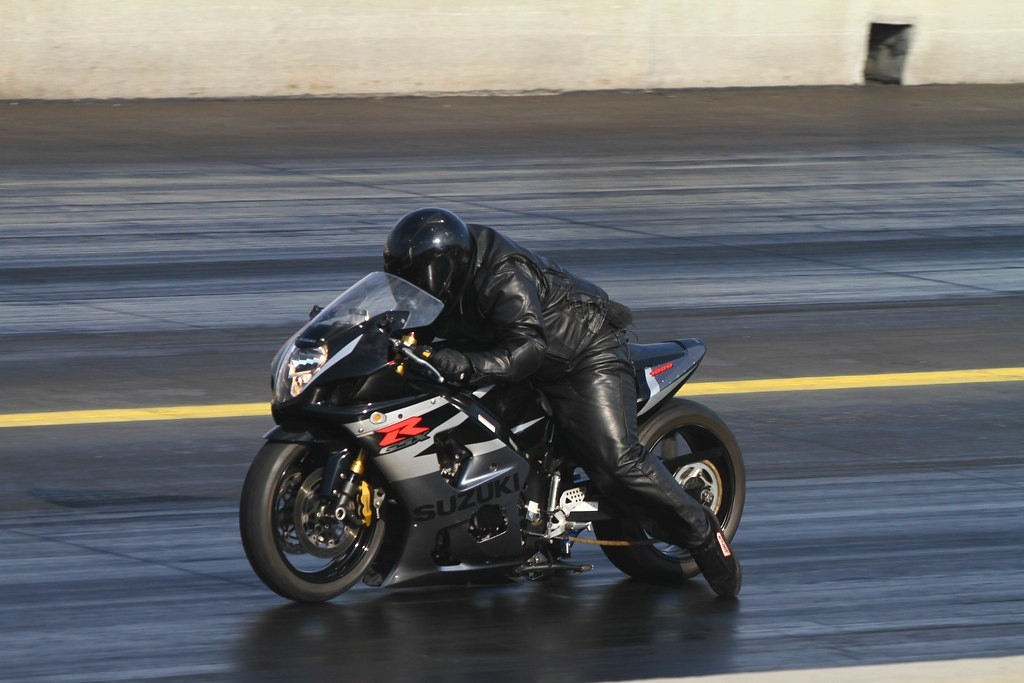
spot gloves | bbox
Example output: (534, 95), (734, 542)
(421, 347), (472, 390)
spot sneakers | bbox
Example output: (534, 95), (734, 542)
(691, 504), (743, 600)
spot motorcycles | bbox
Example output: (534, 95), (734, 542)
(239, 271), (746, 604)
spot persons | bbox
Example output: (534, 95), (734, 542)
(310, 208), (742, 600)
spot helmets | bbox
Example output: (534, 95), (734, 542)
(383, 206), (474, 318)
